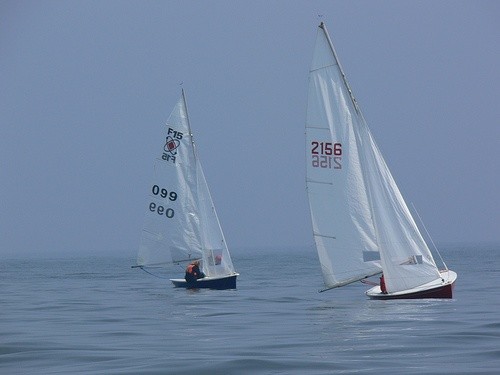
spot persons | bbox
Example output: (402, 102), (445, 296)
(380, 273), (389, 293)
(185, 260), (206, 282)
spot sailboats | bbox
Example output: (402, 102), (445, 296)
(304, 12), (459, 298)
(130, 81), (240, 291)
